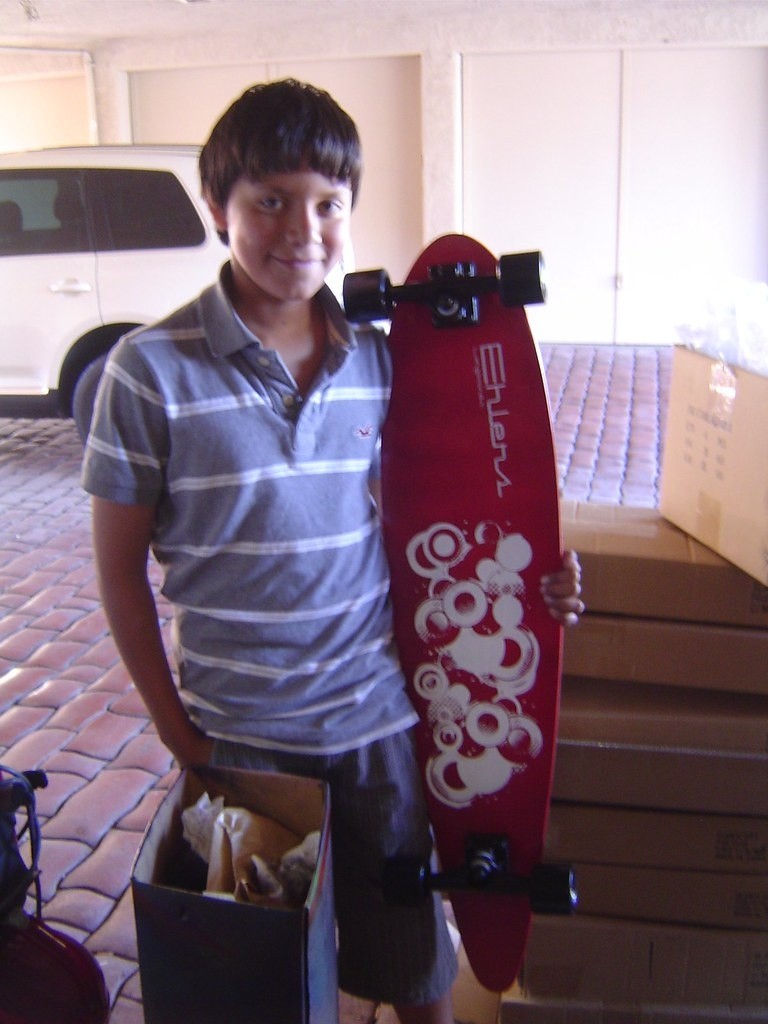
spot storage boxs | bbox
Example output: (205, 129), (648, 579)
(451, 500), (766, 1024)
(130, 767), (339, 1024)
(657, 345), (766, 587)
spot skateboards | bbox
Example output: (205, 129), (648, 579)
(340, 230), (583, 996)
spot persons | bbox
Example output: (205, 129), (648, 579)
(83, 78), (586, 1023)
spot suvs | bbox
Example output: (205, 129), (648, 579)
(1, 144), (357, 448)
